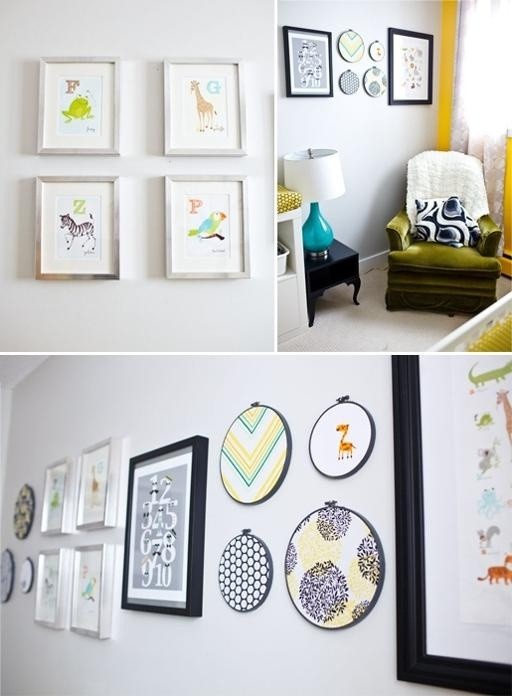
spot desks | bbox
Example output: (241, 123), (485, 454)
(304, 238), (361, 328)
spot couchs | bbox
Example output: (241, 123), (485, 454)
(385, 150), (502, 316)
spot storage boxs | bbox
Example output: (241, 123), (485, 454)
(278, 242), (290, 277)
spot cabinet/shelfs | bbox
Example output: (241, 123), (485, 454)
(278, 208), (310, 348)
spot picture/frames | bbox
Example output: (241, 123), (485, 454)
(0, 436), (123, 640)
(282, 25), (332, 98)
(388, 28), (433, 106)
(36, 55), (121, 157)
(284, 500), (386, 630)
(217, 528), (272, 611)
(391, 356), (512, 694)
(219, 404), (292, 507)
(120, 434), (209, 618)
(163, 59), (249, 158)
(163, 174), (251, 280)
(308, 400), (376, 478)
(34, 175), (120, 280)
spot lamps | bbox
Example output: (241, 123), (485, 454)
(284, 149), (346, 260)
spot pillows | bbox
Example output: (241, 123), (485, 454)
(414, 197), (464, 247)
(460, 205), (481, 247)
